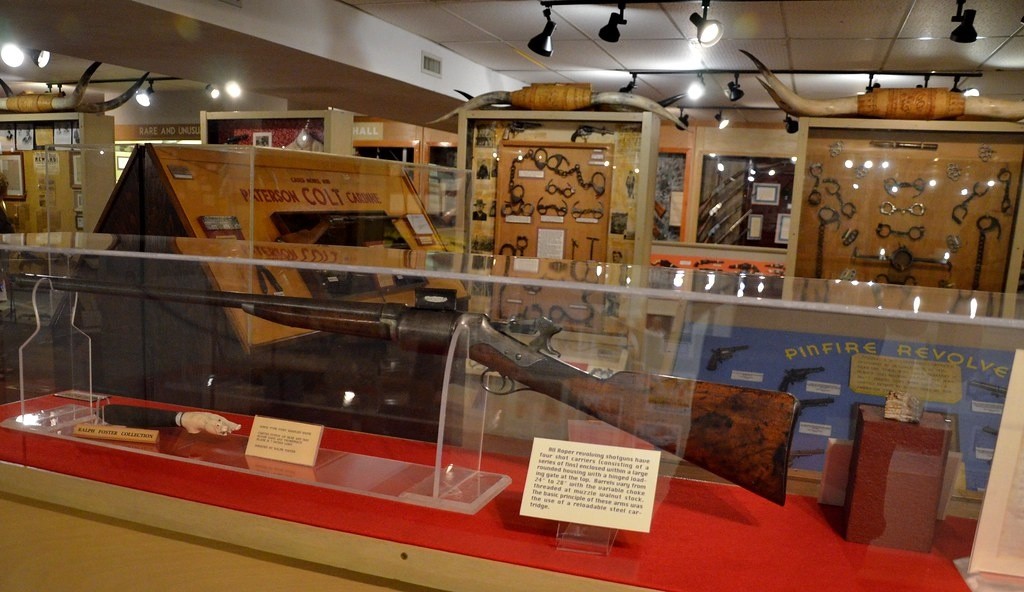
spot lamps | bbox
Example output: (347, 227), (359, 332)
(950, 0), (977, 43)
(619, 75), (638, 95)
(714, 110), (730, 129)
(689, 0), (724, 48)
(599, 3), (628, 44)
(527, 9), (557, 57)
(135, 81), (154, 108)
(782, 113), (798, 135)
(727, 73), (744, 102)
(676, 108), (690, 131)
(206, 83), (219, 98)
(23, 48), (50, 68)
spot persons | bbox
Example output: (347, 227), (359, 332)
(474, 158), (498, 221)
(612, 251), (622, 263)
(101, 404), (243, 436)
(626, 170), (636, 198)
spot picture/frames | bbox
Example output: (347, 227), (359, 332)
(0, 151), (26, 201)
(68, 149), (83, 189)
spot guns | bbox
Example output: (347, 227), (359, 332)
(7, 264), (809, 511)
(696, 168), (760, 245)
